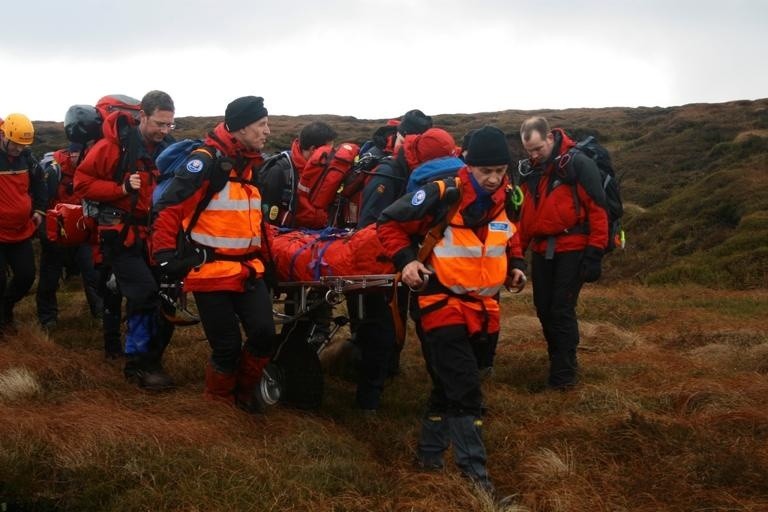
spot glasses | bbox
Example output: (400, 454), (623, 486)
(149, 117), (176, 130)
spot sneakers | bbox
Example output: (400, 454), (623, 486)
(124, 353), (177, 395)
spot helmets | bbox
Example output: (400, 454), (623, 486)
(63, 104), (104, 145)
(0, 113), (34, 145)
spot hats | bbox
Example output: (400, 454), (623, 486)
(224, 95), (268, 133)
(465, 124), (512, 166)
(373, 109), (462, 168)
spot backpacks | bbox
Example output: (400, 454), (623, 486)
(405, 154), (468, 272)
(296, 142), (361, 231)
(92, 225), (147, 251)
(39, 151), (62, 185)
(259, 149), (298, 229)
(559, 133), (624, 222)
(82, 93), (142, 224)
(151, 137), (235, 238)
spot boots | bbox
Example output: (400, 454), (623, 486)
(450, 414), (492, 495)
(414, 409), (450, 475)
(202, 343), (273, 415)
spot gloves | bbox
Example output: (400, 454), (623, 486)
(154, 250), (180, 283)
(574, 243), (605, 283)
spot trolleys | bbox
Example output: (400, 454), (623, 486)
(91, 262), (528, 415)
